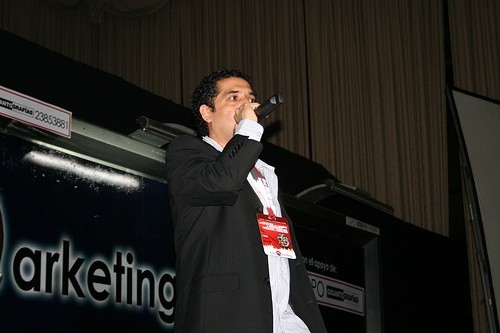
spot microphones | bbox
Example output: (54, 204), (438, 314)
(254, 91), (283, 122)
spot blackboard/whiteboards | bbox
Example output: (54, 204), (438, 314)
(447, 86), (500, 333)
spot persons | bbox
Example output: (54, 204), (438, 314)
(165, 69), (328, 333)
